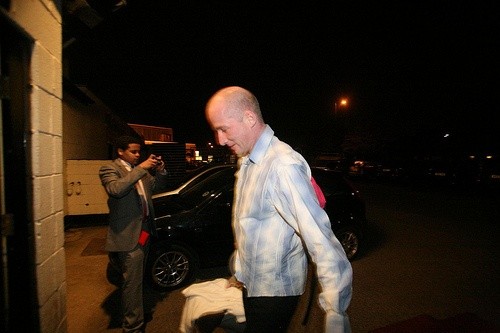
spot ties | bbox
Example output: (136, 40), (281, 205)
(131, 166), (147, 220)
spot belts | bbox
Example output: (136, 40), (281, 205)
(128, 244), (140, 253)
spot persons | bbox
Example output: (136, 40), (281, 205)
(205, 86), (353, 333)
(98, 133), (166, 333)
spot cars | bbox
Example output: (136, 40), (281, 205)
(107, 164), (366, 292)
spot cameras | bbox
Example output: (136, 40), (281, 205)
(153, 156), (162, 166)
